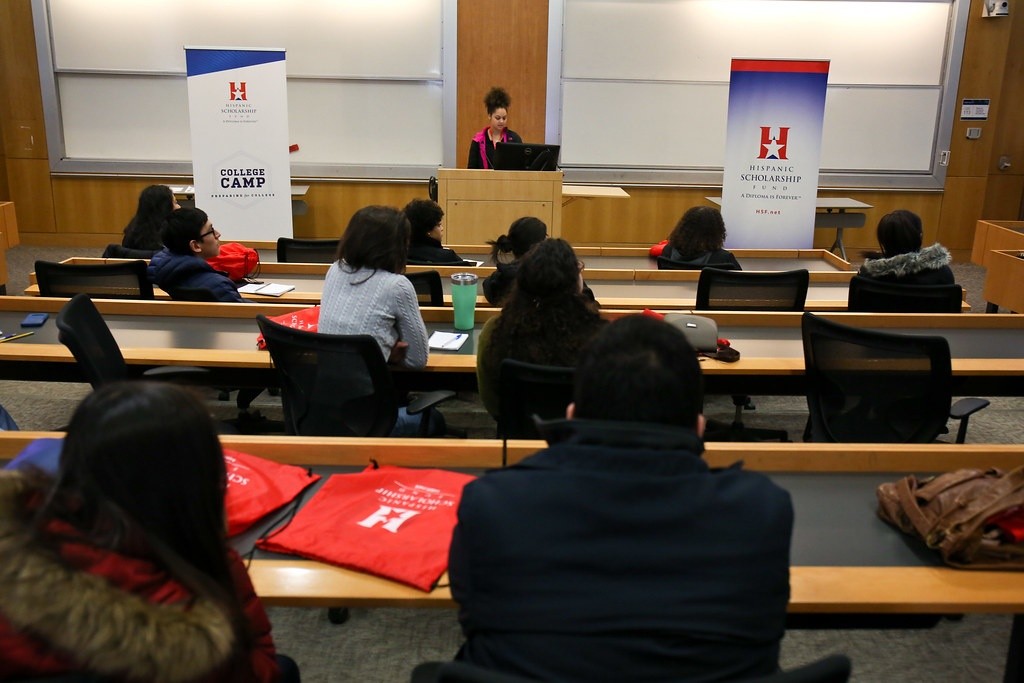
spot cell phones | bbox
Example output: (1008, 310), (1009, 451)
(20, 313), (50, 327)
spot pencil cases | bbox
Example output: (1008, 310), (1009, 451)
(703, 347), (741, 362)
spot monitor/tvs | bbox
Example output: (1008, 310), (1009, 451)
(494, 142), (560, 172)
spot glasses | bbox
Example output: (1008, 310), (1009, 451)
(196, 229), (216, 243)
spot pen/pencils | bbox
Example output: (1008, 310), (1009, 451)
(0, 332), (34, 343)
(442, 335), (461, 347)
(698, 358), (709, 361)
(255, 283), (271, 291)
(0, 334), (16, 340)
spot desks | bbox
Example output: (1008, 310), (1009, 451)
(0, 242), (1024, 683)
(705, 196), (874, 265)
(160, 184), (309, 216)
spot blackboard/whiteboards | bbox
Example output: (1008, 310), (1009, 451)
(30, 0), (972, 194)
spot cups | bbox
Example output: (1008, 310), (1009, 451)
(450, 272), (479, 330)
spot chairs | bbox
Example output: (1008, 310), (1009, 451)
(847, 273), (969, 312)
(407, 264), (447, 306)
(656, 255), (738, 271)
(799, 307), (994, 444)
(255, 312), (468, 439)
(404, 641), (858, 683)
(694, 264), (811, 442)
(276, 235), (342, 263)
(492, 352), (577, 438)
(104, 243), (163, 259)
(29, 250), (164, 300)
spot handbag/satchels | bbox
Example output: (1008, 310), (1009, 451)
(664, 313), (741, 363)
(251, 459), (478, 592)
(220, 444), (322, 542)
(256, 305), (326, 350)
(874, 466), (1024, 573)
(208, 243), (261, 282)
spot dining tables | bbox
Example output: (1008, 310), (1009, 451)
(53, 292), (217, 390)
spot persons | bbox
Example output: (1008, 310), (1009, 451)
(0, 375), (278, 683)
(148, 207), (243, 303)
(857, 210), (954, 287)
(448, 315), (795, 683)
(478, 238), (609, 425)
(318, 203), (446, 438)
(483, 217), (587, 306)
(404, 198), (462, 263)
(467, 88), (522, 169)
(660, 206), (742, 271)
(122, 185), (180, 251)
